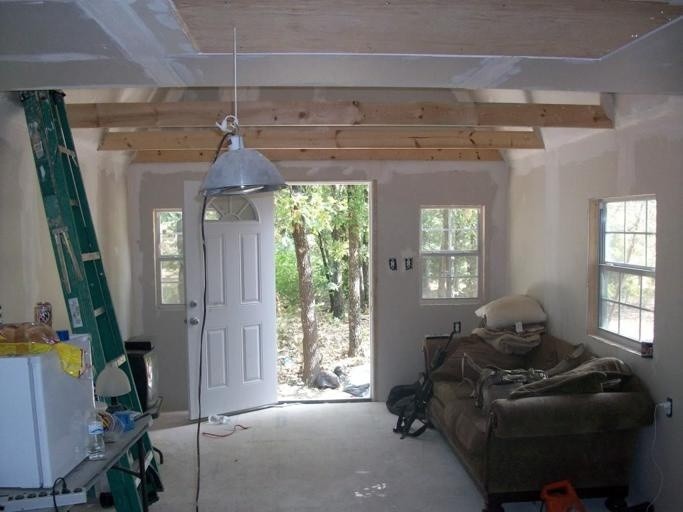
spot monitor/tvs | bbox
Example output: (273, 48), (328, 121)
(127, 348), (160, 410)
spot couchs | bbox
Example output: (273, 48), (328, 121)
(422, 333), (654, 511)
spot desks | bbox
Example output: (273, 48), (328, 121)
(0, 411), (154, 512)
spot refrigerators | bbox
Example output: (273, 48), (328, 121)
(0, 334), (96, 488)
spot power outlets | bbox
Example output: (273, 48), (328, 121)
(662, 400), (673, 416)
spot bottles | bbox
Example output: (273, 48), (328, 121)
(86, 409), (106, 460)
(208, 414), (231, 424)
(33, 296), (52, 327)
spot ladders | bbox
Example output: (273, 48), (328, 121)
(20, 88), (163, 512)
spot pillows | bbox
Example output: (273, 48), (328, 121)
(474, 294), (549, 328)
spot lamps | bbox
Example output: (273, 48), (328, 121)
(200, 26), (289, 198)
(95, 366), (131, 414)
